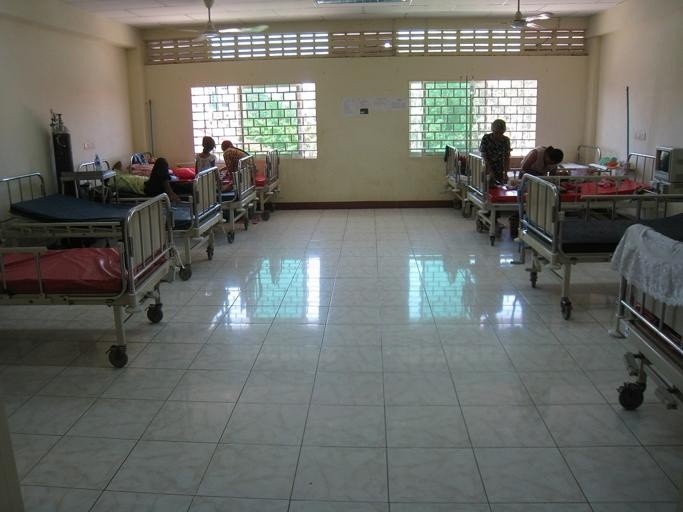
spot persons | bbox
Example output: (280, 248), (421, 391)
(479, 119), (512, 225)
(517, 145), (564, 187)
(194, 136), (223, 191)
(99, 157), (235, 203)
(219, 141), (256, 182)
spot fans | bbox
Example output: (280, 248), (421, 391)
(174, 0), (269, 45)
(479, 1), (555, 32)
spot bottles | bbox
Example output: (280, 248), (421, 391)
(94, 154), (102, 171)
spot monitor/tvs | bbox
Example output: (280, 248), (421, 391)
(654, 146), (683, 182)
